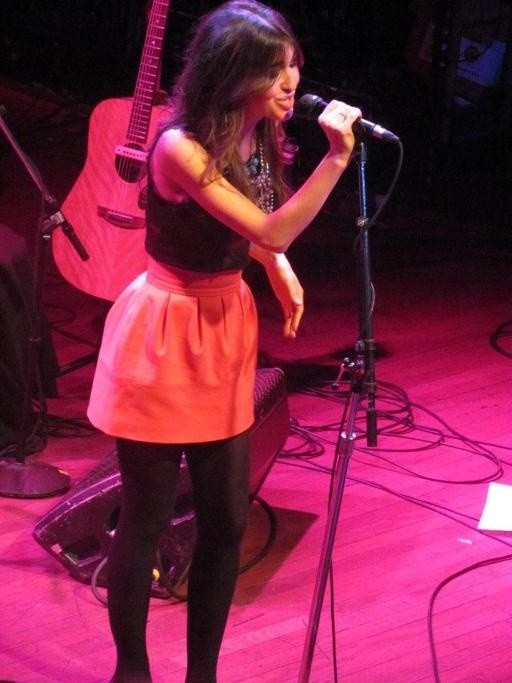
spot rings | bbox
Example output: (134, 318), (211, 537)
(339, 111), (345, 120)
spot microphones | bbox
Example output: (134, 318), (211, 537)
(297, 93), (400, 143)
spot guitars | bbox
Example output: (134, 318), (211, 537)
(51, 0), (175, 301)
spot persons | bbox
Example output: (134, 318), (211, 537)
(87, 3), (361, 683)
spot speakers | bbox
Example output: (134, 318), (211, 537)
(33, 367), (291, 600)
